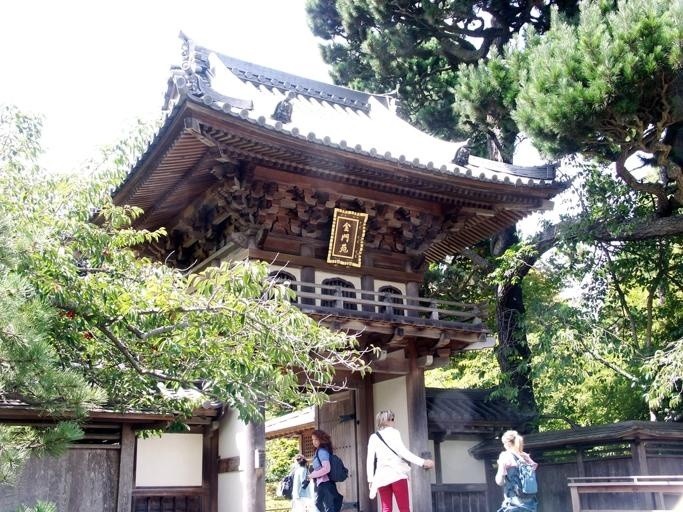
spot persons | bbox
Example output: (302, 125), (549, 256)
(495, 430), (538, 512)
(366, 410), (433, 512)
(277, 430), (347, 512)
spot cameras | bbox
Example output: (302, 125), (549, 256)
(302, 479), (310, 488)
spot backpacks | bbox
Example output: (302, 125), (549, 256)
(315, 447), (349, 482)
(504, 451), (538, 497)
